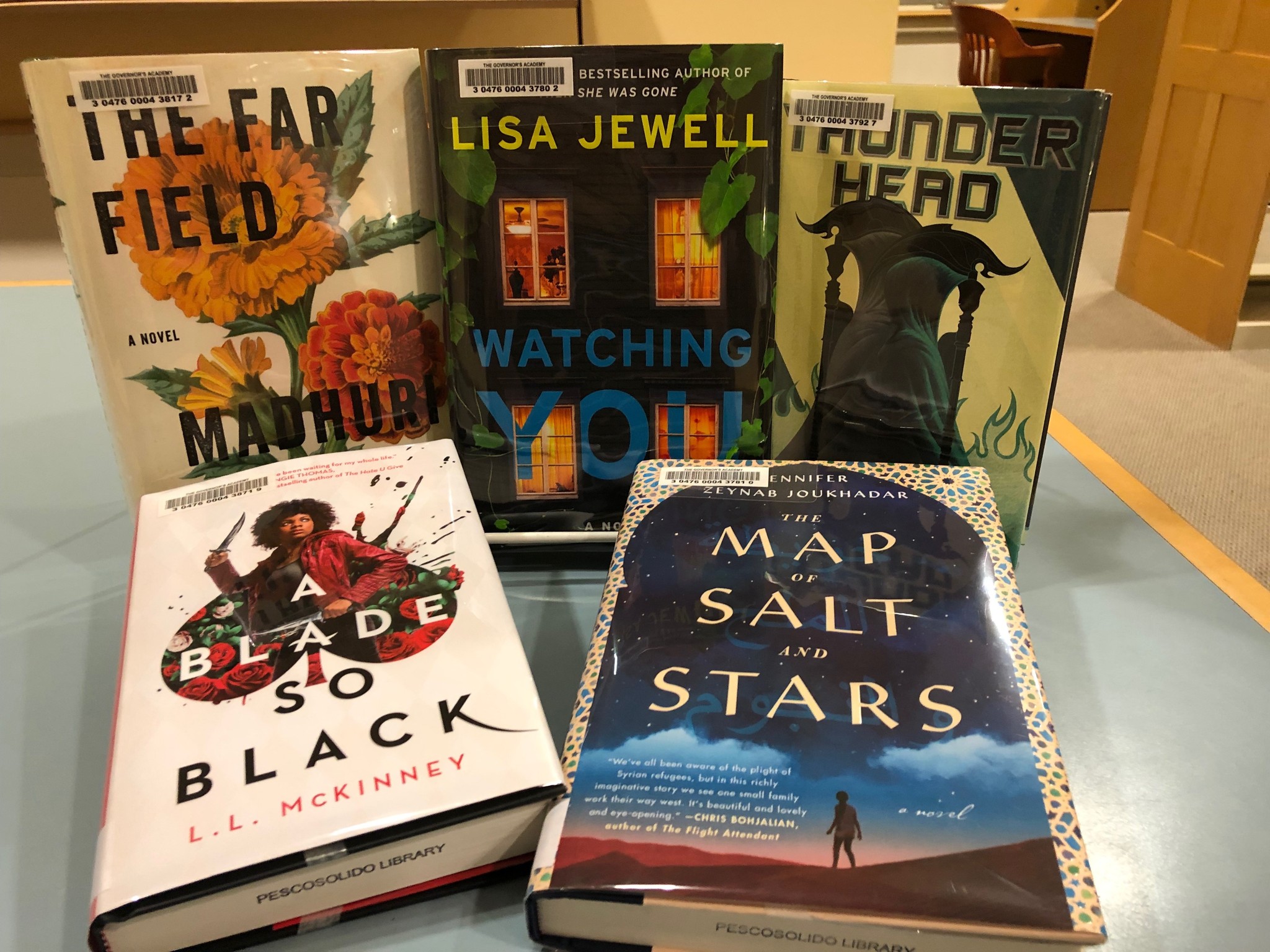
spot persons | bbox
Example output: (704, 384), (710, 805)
(204, 498), (408, 683)
(17, 46), (458, 503)
(826, 791), (863, 875)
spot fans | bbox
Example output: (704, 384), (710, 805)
(504, 207), (560, 229)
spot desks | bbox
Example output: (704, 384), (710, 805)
(1001, 0), (1172, 212)
(1, 283), (1269, 951)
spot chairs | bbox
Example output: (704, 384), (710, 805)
(947, 0), (1063, 89)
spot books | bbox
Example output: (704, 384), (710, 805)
(424, 42), (781, 572)
(768, 77), (1114, 575)
(86, 437), (573, 952)
(524, 462), (1107, 951)
